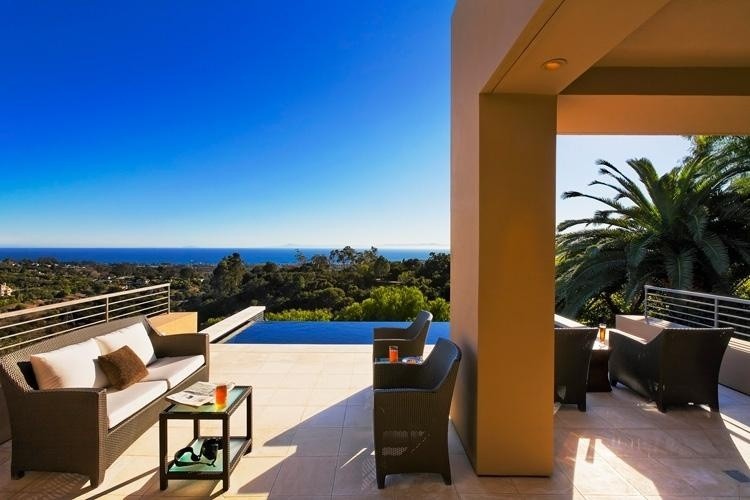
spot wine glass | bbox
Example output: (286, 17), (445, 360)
(598, 324), (607, 348)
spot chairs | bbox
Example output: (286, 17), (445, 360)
(555, 328), (598, 412)
(608, 327), (735, 412)
(374, 310), (433, 356)
(374, 337), (461, 490)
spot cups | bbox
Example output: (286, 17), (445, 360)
(216, 385), (228, 408)
(389, 345), (399, 363)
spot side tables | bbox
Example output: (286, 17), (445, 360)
(587, 349), (613, 392)
(374, 357), (426, 365)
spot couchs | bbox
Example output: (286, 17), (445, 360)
(0, 315), (210, 489)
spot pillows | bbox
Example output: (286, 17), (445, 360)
(29, 320), (158, 388)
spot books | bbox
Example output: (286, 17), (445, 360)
(166, 381), (235, 408)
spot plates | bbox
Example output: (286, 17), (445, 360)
(401, 356), (424, 364)
